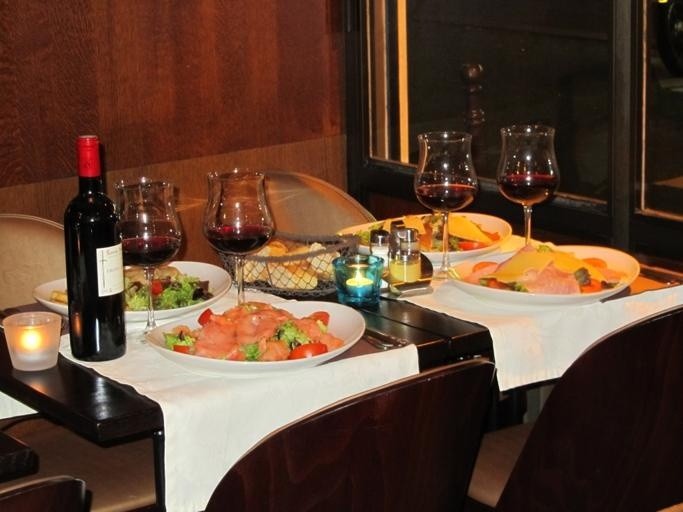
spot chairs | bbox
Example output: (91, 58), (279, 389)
(203, 360), (499, 510)
(459, 304), (682, 510)
(262, 171), (378, 241)
(0, 211), (70, 310)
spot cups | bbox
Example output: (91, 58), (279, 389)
(3, 312), (61, 372)
(331, 255), (383, 308)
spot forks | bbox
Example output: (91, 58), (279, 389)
(363, 335), (388, 351)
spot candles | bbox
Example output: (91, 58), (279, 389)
(347, 273), (373, 297)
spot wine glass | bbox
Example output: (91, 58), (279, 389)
(202, 170), (278, 308)
(115, 179), (182, 334)
(497, 127), (512, 201)
(415, 133), (479, 279)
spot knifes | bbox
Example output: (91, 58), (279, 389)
(366, 328), (407, 346)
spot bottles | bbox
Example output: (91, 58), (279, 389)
(63, 136), (127, 362)
(369, 230), (389, 278)
(389, 228), (421, 283)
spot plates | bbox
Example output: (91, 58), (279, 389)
(32, 261), (231, 322)
(337, 212), (511, 266)
(145, 301), (365, 373)
(447, 245), (640, 303)
